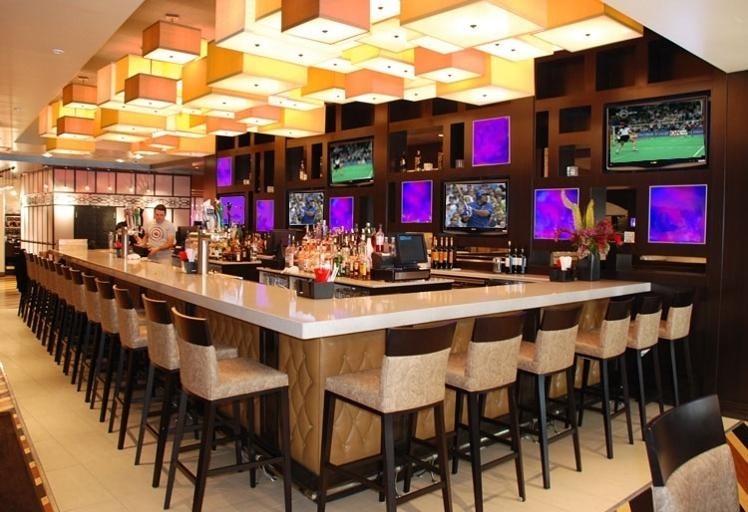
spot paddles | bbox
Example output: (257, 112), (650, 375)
(604, 420), (748, 511)
(0, 410), (48, 512)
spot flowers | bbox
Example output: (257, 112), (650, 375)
(554, 190), (628, 261)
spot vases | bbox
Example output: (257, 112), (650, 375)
(575, 246), (601, 282)
(554, 190), (628, 261)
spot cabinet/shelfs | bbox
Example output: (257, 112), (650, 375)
(399, 151), (407, 173)
(284, 217), (395, 281)
(198, 238), (207, 275)
(122, 235), (129, 259)
(298, 159), (307, 181)
(243, 176), (249, 185)
(414, 150), (422, 172)
(504, 240), (526, 274)
(427, 235), (456, 270)
(221, 223), (273, 262)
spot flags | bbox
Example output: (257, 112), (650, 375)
(575, 246), (601, 282)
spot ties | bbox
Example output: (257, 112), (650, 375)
(395, 233), (427, 271)
(605, 95), (708, 172)
(442, 179), (507, 237)
(329, 137), (373, 186)
(287, 189), (324, 227)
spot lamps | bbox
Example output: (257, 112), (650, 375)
(641, 394), (740, 512)
(37, 0), (644, 162)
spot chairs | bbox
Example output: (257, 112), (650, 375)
(161, 304), (293, 512)
(134, 293), (243, 490)
(314, 318), (459, 511)
(561, 296), (635, 459)
(641, 394), (740, 512)
(633, 294), (695, 420)
(477, 300), (584, 490)
(108, 284), (176, 449)
(16, 254), (143, 422)
(403, 311), (530, 509)
(596, 295), (664, 440)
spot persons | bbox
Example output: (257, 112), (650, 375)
(609, 102), (702, 153)
(329, 142), (372, 170)
(142, 205), (174, 265)
(288, 192), (324, 226)
(445, 183), (506, 228)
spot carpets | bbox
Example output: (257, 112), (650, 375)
(0, 410), (48, 512)
(604, 420), (748, 511)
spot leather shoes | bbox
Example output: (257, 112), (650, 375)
(461, 204), (473, 218)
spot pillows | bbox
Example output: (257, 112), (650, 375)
(306, 197), (314, 202)
(476, 190), (489, 197)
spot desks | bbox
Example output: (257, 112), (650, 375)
(254, 263), (456, 300)
(429, 268), (551, 288)
(200, 253), (287, 280)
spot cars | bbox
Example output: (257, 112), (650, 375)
(423, 163), (434, 170)
(438, 152), (443, 170)
(455, 159), (464, 168)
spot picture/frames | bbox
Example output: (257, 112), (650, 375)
(254, 263), (456, 300)
